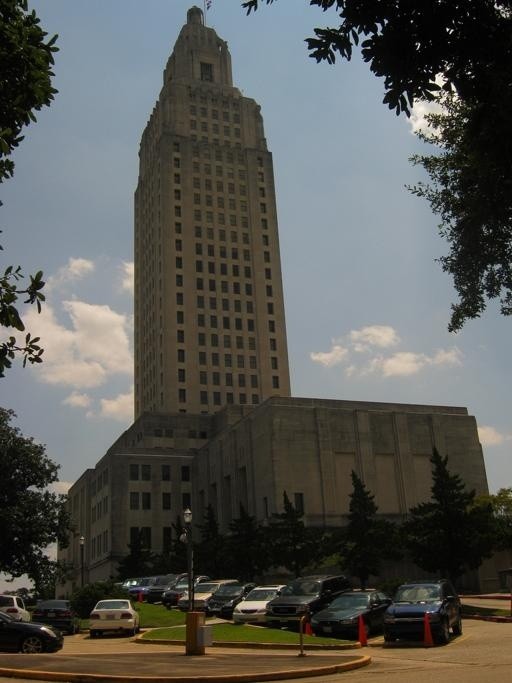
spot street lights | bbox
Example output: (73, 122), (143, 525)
(183, 508), (193, 612)
(79, 536), (85, 587)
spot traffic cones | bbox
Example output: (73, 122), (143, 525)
(422, 613), (434, 646)
(358, 612), (367, 646)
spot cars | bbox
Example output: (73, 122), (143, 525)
(87, 599), (140, 638)
(0, 595), (82, 653)
